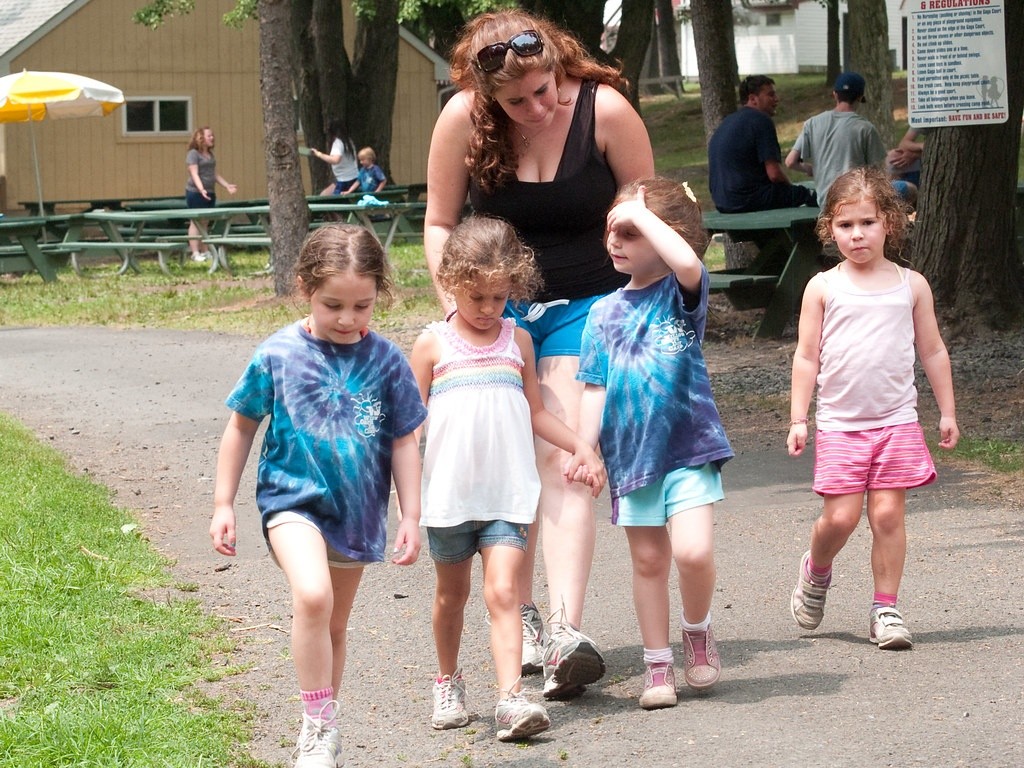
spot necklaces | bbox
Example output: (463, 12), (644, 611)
(514, 126), (545, 148)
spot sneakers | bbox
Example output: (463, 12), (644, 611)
(191, 250), (213, 261)
(639, 663), (679, 708)
(431, 666), (483, 729)
(495, 675), (551, 741)
(870, 606), (914, 650)
(790, 551), (833, 629)
(542, 608), (606, 698)
(682, 622), (721, 688)
(290, 699), (345, 768)
(484, 601), (545, 675)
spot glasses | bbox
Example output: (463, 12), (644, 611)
(475, 29), (545, 72)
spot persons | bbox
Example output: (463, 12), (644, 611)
(310, 121), (358, 223)
(884, 110), (926, 188)
(422, 6), (651, 700)
(788, 166), (962, 653)
(706, 75), (817, 274)
(565, 176), (734, 707)
(185, 127), (237, 262)
(209, 223), (429, 768)
(340, 147), (393, 219)
(784, 71), (918, 212)
(399, 218), (612, 744)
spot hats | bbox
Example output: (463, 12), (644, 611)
(834, 72), (867, 104)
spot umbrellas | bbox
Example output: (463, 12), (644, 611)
(0, 67), (125, 244)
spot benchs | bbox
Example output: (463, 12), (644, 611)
(0, 197), (473, 256)
(708, 274), (779, 290)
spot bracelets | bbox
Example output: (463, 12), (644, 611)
(789, 419), (808, 427)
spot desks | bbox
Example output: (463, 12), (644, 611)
(704, 183), (867, 327)
(0, 181), (427, 287)
